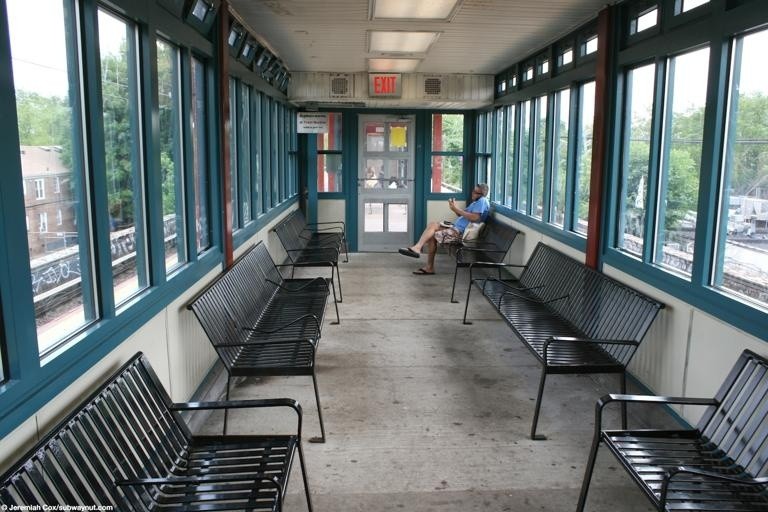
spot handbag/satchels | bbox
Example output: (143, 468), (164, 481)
(462, 221), (485, 248)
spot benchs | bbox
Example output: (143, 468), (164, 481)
(463, 242), (666, 440)
(440, 216), (520, 303)
(0, 351), (314, 512)
(187, 240), (340, 443)
(268, 208), (348, 302)
(576, 348), (768, 512)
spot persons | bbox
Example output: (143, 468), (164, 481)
(398, 183), (491, 274)
(364, 164), (407, 189)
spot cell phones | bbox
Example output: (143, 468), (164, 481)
(476, 195), (482, 201)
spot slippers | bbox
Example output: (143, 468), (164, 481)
(398, 247), (419, 258)
(412, 268), (434, 275)
